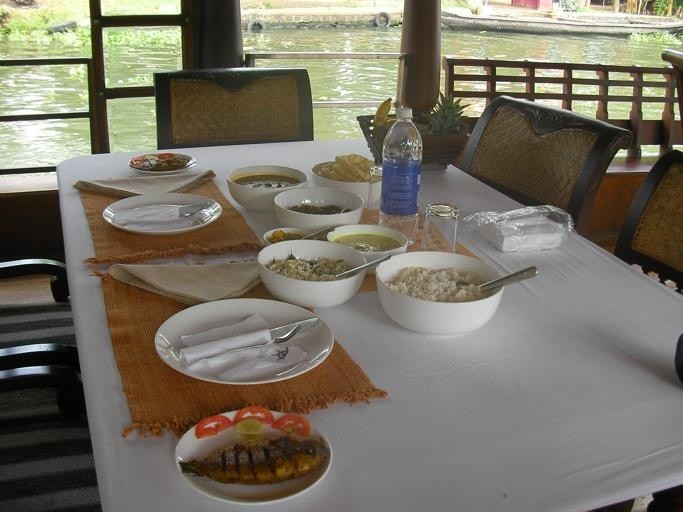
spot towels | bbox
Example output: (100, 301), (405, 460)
(72, 167), (218, 202)
(105, 261), (262, 308)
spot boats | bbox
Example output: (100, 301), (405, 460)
(441, 5), (683, 37)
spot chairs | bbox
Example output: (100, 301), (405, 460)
(614, 146), (683, 296)
(455, 92), (632, 241)
(151, 66), (315, 152)
(660, 48), (682, 132)
(0, 256), (76, 368)
(0, 364), (106, 512)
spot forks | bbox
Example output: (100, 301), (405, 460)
(266, 324), (302, 347)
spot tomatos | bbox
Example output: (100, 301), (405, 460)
(196, 404), (310, 437)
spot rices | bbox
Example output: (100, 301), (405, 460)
(386, 267), (484, 301)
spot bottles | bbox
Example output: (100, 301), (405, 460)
(380, 106), (423, 251)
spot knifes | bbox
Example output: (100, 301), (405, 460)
(268, 316), (319, 344)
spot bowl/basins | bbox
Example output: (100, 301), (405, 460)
(274, 187), (366, 231)
(375, 250), (506, 336)
(257, 239), (370, 307)
(262, 226), (319, 245)
(422, 202), (458, 252)
(225, 164), (307, 211)
(313, 162), (381, 210)
(327, 225), (410, 277)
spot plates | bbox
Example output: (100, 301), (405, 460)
(99, 192), (221, 237)
(128, 149), (197, 175)
(153, 295), (333, 387)
(173, 406), (334, 508)
